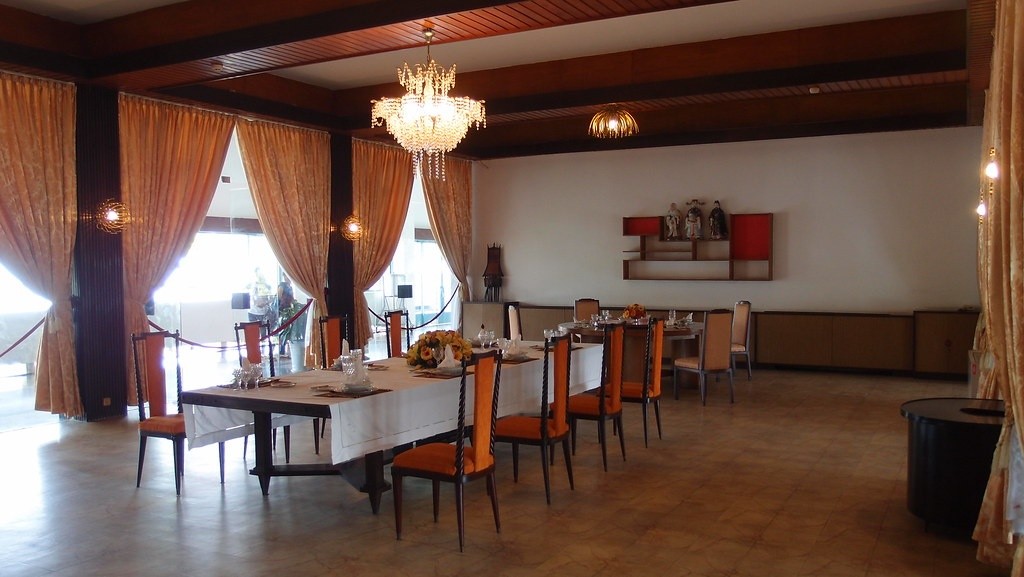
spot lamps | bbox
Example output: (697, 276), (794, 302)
(340, 213), (365, 241)
(482, 243), (505, 302)
(588, 97), (640, 139)
(370, 27), (486, 182)
(95, 196), (133, 235)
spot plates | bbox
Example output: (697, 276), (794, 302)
(327, 389), (382, 397)
(260, 377), (280, 386)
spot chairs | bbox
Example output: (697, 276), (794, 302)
(132, 297), (752, 554)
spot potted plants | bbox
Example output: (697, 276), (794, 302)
(280, 302), (309, 373)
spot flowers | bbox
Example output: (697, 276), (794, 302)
(623, 303), (647, 320)
(406, 329), (473, 369)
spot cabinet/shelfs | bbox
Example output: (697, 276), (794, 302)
(622, 213), (773, 281)
(914, 306), (983, 378)
(461, 300), (520, 340)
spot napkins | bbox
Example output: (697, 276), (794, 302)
(437, 344), (462, 369)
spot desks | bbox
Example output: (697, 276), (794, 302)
(900, 397), (1006, 539)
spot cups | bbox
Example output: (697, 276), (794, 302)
(340, 349), (362, 382)
(543, 328), (564, 342)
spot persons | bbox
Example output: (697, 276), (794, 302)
(665, 203), (683, 240)
(248, 269), (272, 354)
(709, 201), (726, 240)
(685, 200), (705, 240)
(279, 273), (294, 359)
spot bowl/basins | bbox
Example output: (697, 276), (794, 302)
(343, 382), (370, 391)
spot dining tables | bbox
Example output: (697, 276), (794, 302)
(558, 319), (704, 344)
(180, 339), (604, 516)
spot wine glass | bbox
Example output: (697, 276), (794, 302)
(668, 310), (675, 328)
(590, 314), (599, 330)
(230, 365), (263, 392)
(497, 337), (507, 358)
(478, 331), (495, 351)
(602, 310), (609, 322)
